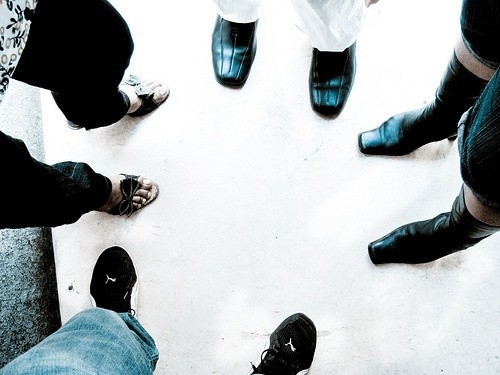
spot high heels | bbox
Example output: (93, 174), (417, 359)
(107, 173), (159, 218)
(66, 74), (170, 130)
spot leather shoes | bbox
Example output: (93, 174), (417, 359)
(210, 13), (259, 86)
(309, 39), (358, 116)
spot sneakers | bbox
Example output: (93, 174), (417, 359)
(250, 312), (317, 375)
(90, 245), (139, 321)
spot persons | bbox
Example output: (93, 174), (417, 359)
(1, 0), (170, 230)
(355, 2), (500, 265)
(210, 0), (382, 116)
(0, 244), (319, 375)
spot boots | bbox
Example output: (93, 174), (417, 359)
(358, 45), (490, 157)
(367, 182), (500, 265)
